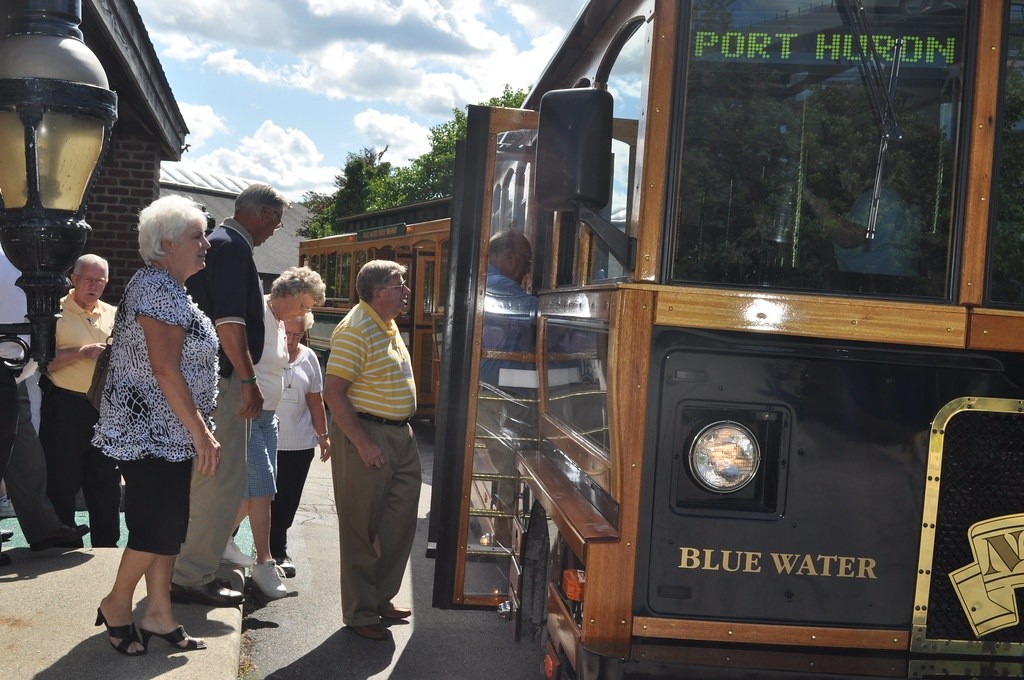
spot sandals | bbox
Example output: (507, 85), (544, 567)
(95, 608), (148, 656)
(140, 625), (208, 650)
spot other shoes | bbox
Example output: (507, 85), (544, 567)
(0, 552), (11, 566)
(0, 530), (14, 541)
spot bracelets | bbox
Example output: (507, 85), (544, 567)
(241, 375), (256, 384)
(317, 432), (327, 438)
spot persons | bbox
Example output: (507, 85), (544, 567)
(220, 266), (327, 599)
(476, 230), (539, 548)
(90, 193), (222, 656)
(269, 311), (331, 578)
(38, 254), (122, 548)
(169, 183), (286, 607)
(323, 259), (422, 640)
(0, 241), (89, 566)
(801, 159), (921, 278)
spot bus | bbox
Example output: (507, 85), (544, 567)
(421, 0), (1024, 679)
(295, 196), (454, 424)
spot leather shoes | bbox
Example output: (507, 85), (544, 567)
(356, 626), (388, 639)
(30, 524), (91, 551)
(382, 603), (412, 619)
(171, 577), (243, 607)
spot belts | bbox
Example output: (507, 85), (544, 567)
(355, 412), (412, 427)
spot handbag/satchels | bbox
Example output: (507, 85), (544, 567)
(86, 345), (111, 413)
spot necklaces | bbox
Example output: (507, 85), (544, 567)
(269, 301), (275, 315)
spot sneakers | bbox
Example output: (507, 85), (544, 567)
(220, 536), (254, 568)
(275, 557), (296, 578)
(251, 554), (287, 598)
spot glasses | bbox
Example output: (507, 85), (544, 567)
(274, 211), (285, 232)
(72, 273), (106, 288)
(510, 248), (535, 265)
(377, 280), (407, 293)
(300, 305), (311, 315)
